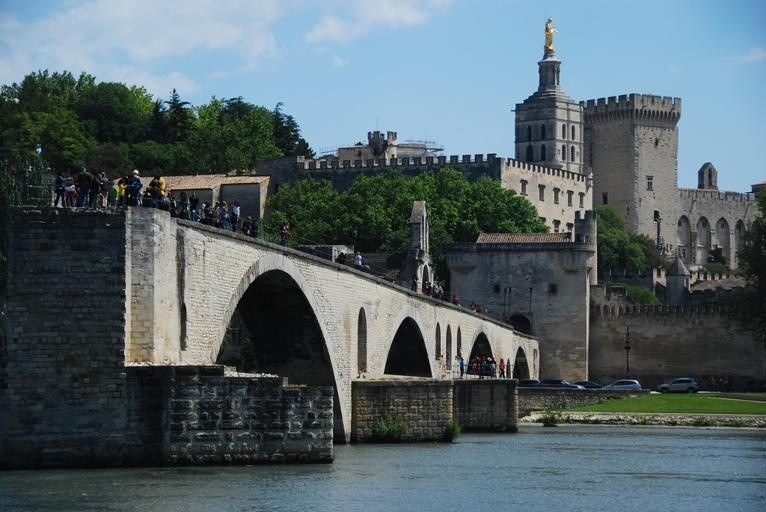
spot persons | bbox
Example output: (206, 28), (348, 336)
(335, 251), (368, 270)
(470, 301), (482, 313)
(280, 221), (290, 240)
(451, 291), (460, 305)
(54, 167), (258, 238)
(459, 357), (465, 379)
(468, 356), (496, 379)
(499, 359), (505, 378)
(411, 271), (418, 292)
(545, 17), (556, 45)
(423, 279), (444, 299)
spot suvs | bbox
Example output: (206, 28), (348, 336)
(656, 378), (698, 394)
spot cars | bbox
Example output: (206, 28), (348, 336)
(600, 379), (642, 391)
(518, 378), (602, 390)
